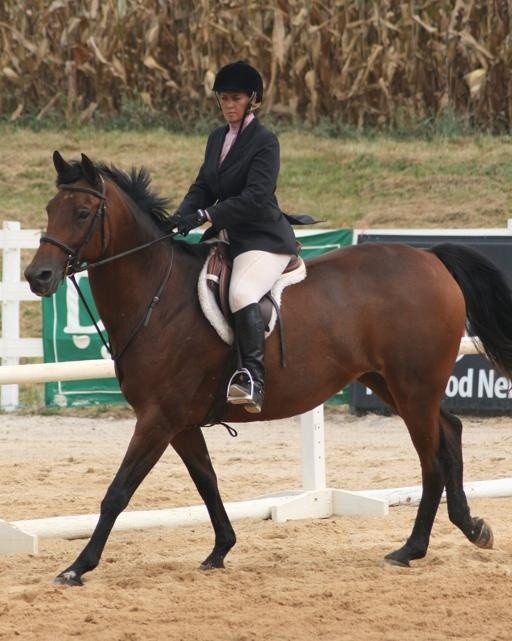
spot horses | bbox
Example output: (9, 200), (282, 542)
(24, 152), (512, 586)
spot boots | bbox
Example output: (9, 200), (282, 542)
(228, 303), (267, 414)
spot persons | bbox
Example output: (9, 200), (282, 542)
(171, 61), (324, 414)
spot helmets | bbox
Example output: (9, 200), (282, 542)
(211, 61), (263, 102)
